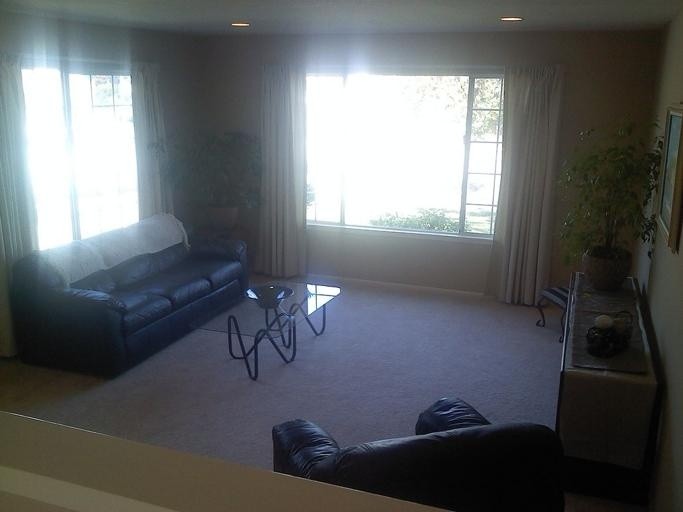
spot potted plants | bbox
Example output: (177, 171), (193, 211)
(548, 109), (670, 292)
(148, 123), (264, 229)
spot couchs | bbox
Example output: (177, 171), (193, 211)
(9, 212), (248, 379)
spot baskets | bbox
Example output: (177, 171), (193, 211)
(588, 311), (633, 358)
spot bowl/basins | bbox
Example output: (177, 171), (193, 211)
(243, 287), (295, 309)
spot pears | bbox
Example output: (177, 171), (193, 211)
(595, 316), (610, 327)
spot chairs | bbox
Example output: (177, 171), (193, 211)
(271, 396), (570, 511)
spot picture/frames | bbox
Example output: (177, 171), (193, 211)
(652, 107), (683, 255)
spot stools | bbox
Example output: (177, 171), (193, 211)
(535, 287), (570, 343)
